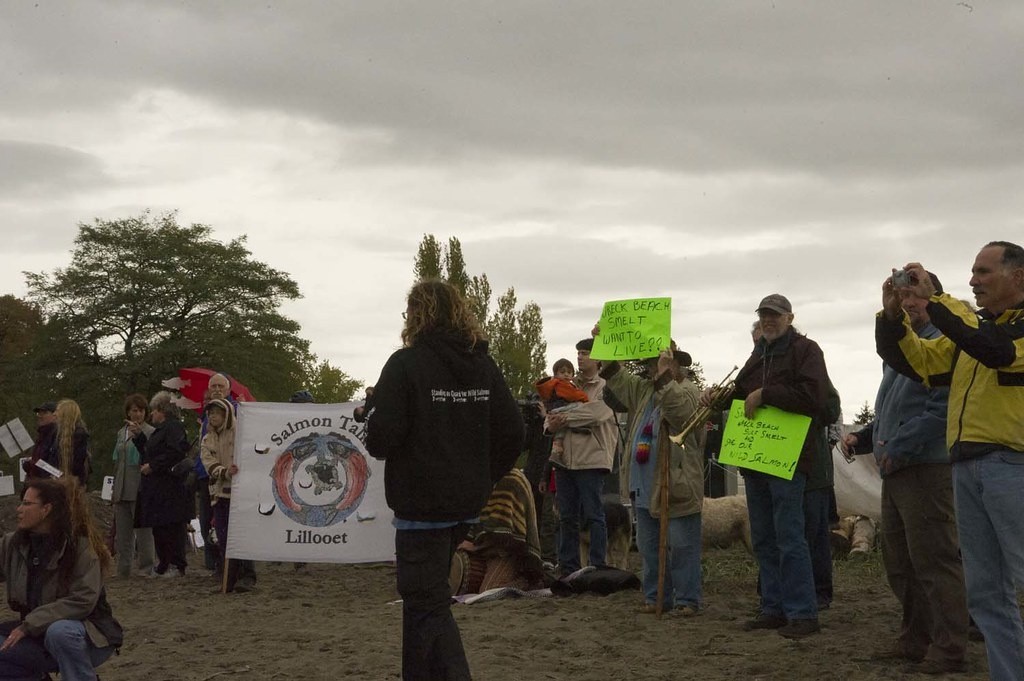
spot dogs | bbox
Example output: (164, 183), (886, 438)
(542, 494), (631, 571)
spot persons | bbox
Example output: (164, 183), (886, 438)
(0, 478), (124, 681)
(19, 375), (258, 593)
(517, 294), (844, 640)
(273, 390), (314, 576)
(841, 241), (1024, 681)
(354, 280), (525, 681)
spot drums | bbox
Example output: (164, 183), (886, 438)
(449, 548), (488, 596)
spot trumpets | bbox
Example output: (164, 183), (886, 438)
(668, 365), (739, 451)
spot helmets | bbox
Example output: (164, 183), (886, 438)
(291, 391), (314, 402)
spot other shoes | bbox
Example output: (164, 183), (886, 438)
(778, 620), (821, 639)
(161, 566), (186, 579)
(876, 642), (921, 661)
(742, 613), (782, 629)
(668, 605), (695, 617)
(905, 656), (965, 673)
(549, 454), (566, 470)
(636, 603), (664, 614)
(235, 578), (254, 592)
(146, 568), (163, 578)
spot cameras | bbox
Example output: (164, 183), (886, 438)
(517, 396), (540, 412)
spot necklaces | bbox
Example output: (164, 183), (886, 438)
(29, 533), (59, 566)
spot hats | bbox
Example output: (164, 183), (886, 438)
(633, 339), (693, 368)
(33, 402), (56, 412)
(754, 294), (791, 314)
(204, 401), (226, 410)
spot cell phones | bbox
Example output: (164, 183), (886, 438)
(892, 271), (910, 288)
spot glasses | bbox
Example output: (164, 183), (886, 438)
(16, 500), (49, 507)
(36, 411), (49, 418)
(756, 310), (782, 319)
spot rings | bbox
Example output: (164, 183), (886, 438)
(7, 635), (15, 640)
